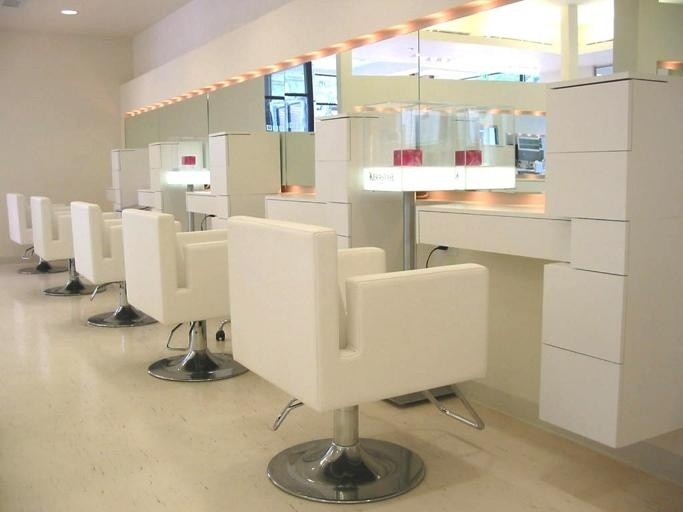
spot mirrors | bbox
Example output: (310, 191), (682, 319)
(123, 1), (615, 212)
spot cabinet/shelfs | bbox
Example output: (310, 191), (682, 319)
(104, 147), (136, 212)
(265, 112), (404, 273)
(136, 140), (190, 231)
(185, 130), (251, 230)
(415, 72), (683, 451)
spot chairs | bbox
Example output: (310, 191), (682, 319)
(30, 196), (106, 296)
(122, 208), (249, 383)
(225, 214), (490, 505)
(6, 192), (69, 275)
(70, 200), (158, 327)
